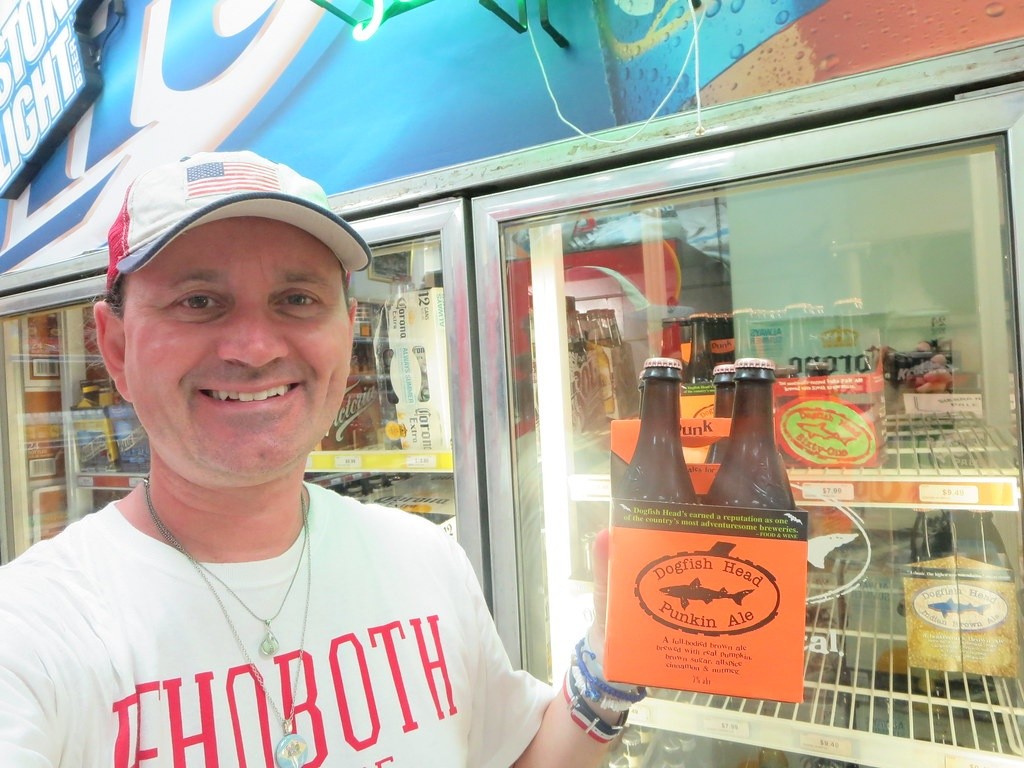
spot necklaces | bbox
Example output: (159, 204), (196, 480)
(142, 473), (313, 768)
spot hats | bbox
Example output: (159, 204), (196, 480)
(106, 151), (373, 292)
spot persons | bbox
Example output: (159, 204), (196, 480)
(0, 150), (648, 768)
(900, 338), (953, 468)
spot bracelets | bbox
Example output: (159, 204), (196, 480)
(562, 631), (647, 744)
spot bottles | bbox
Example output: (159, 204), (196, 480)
(661, 298), (875, 380)
(303, 472), (407, 499)
(622, 356), (795, 513)
(317, 339), (381, 450)
(565, 301), (622, 347)
